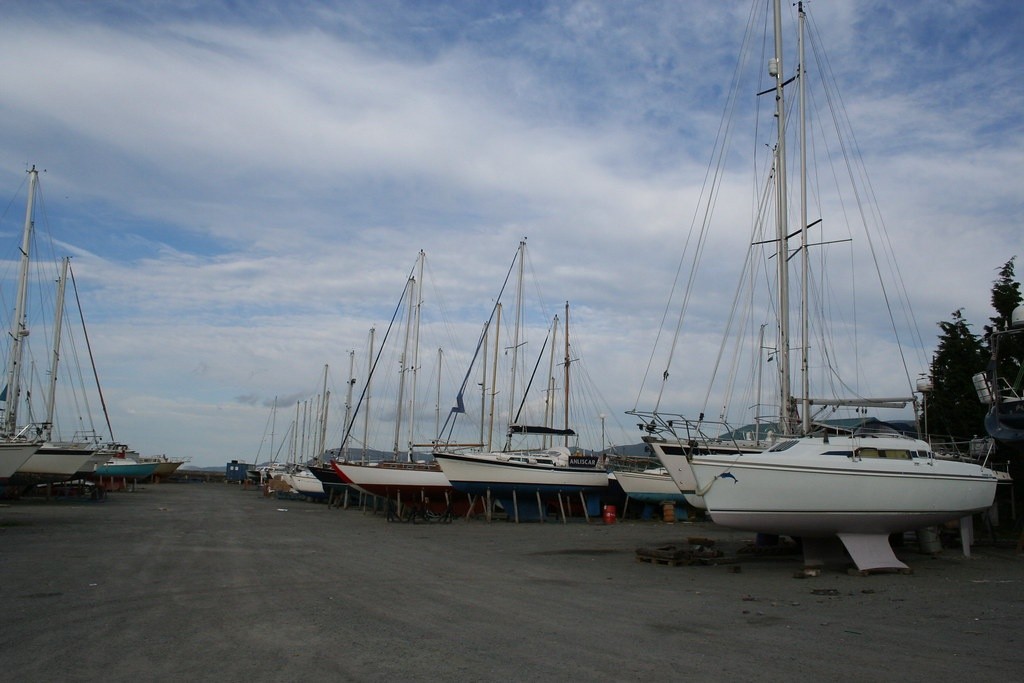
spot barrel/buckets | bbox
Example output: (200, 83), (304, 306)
(604, 505), (616, 524)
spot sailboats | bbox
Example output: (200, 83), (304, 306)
(243, 29), (1009, 533)
(0, 158), (193, 502)
